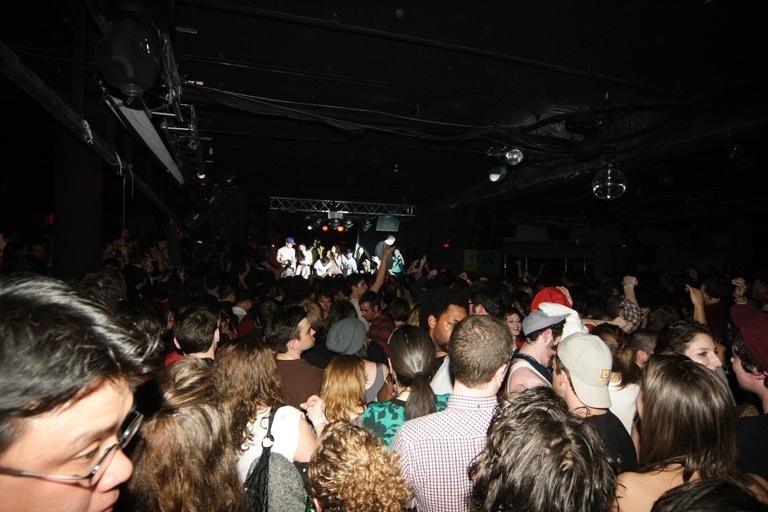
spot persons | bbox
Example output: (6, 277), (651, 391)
(0, 222), (768, 512)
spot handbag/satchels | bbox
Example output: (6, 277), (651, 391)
(244, 449), (272, 512)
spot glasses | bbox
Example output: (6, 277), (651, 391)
(0, 411), (146, 488)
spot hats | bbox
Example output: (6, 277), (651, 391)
(325, 317), (368, 355)
(529, 286), (573, 312)
(557, 333), (616, 410)
(728, 302), (768, 376)
(520, 309), (571, 337)
(286, 237), (296, 245)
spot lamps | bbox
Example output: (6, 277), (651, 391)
(487, 140), (524, 166)
(590, 143), (627, 201)
(304, 212), (354, 234)
(376, 214), (400, 232)
